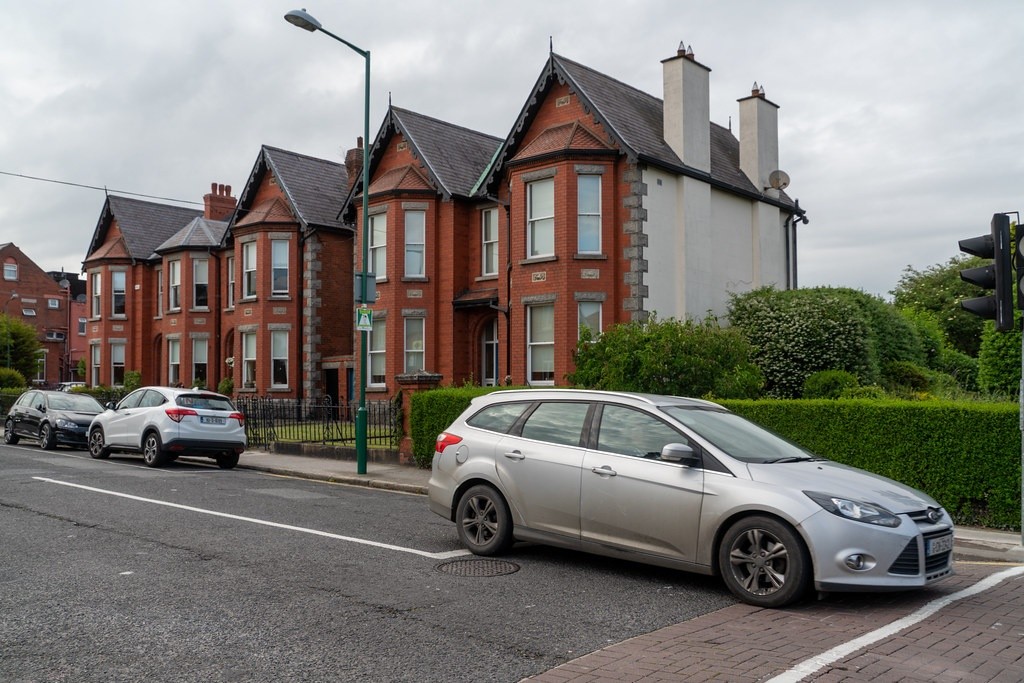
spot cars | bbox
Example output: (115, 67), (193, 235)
(85, 385), (248, 469)
(3, 389), (110, 450)
(425, 387), (955, 606)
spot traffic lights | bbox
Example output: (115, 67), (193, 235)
(957, 212), (1013, 332)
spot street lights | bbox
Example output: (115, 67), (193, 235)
(283, 7), (371, 477)
